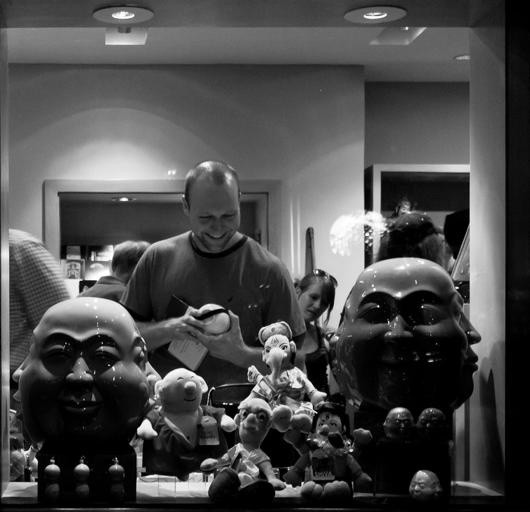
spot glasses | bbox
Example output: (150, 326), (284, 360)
(313, 268), (338, 290)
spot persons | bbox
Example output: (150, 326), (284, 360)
(376, 211), (453, 272)
(119, 158), (306, 382)
(9, 257), (483, 505)
(74, 238), (151, 300)
(378, 199), (410, 249)
(8, 226), (74, 455)
